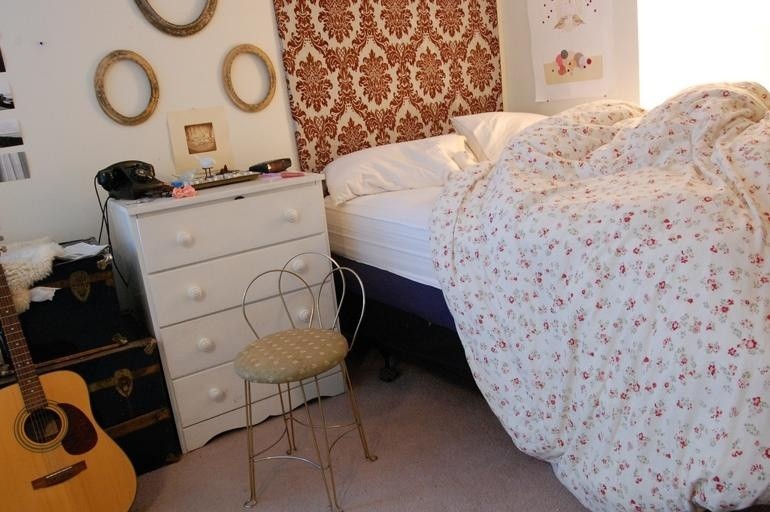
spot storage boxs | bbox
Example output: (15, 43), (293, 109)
(0, 238), (183, 478)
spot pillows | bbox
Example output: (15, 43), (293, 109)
(321, 111), (549, 208)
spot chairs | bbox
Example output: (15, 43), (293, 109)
(234, 252), (380, 512)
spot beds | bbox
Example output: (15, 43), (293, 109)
(323, 112), (488, 396)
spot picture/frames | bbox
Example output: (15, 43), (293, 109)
(165, 106), (233, 179)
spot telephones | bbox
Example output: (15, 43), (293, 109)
(97, 161), (163, 200)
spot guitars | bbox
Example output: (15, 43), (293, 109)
(0, 236), (137, 512)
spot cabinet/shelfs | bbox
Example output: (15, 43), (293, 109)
(103, 169), (348, 454)
